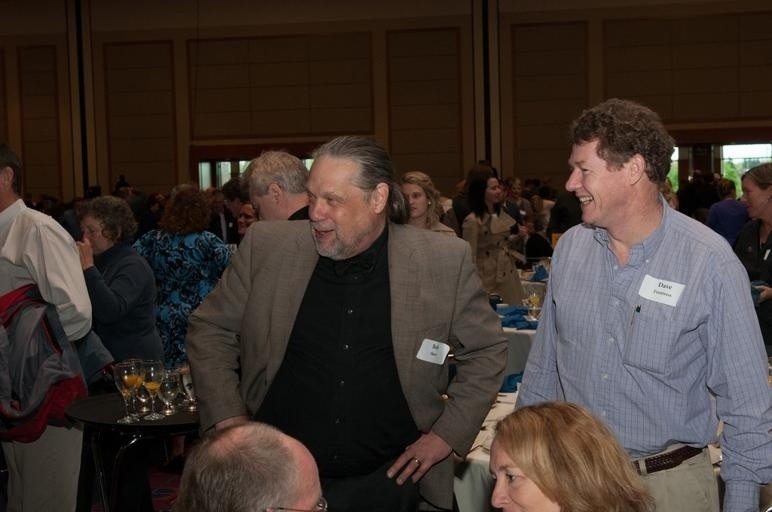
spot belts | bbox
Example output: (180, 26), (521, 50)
(628, 445), (709, 475)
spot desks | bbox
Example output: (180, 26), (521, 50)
(65, 394), (198, 511)
(453, 257), (554, 511)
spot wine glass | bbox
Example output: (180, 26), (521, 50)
(113, 357), (198, 427)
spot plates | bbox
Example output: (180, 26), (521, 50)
(482, 438), (494, 450)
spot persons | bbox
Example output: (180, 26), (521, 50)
(1, 138), (97, 512)
(30, 97), (772, 512)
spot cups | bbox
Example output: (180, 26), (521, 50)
(526, 288), (544, 322)
(489, 293), (509, 312)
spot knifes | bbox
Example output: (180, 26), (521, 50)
(496, 398), (515, 406)
